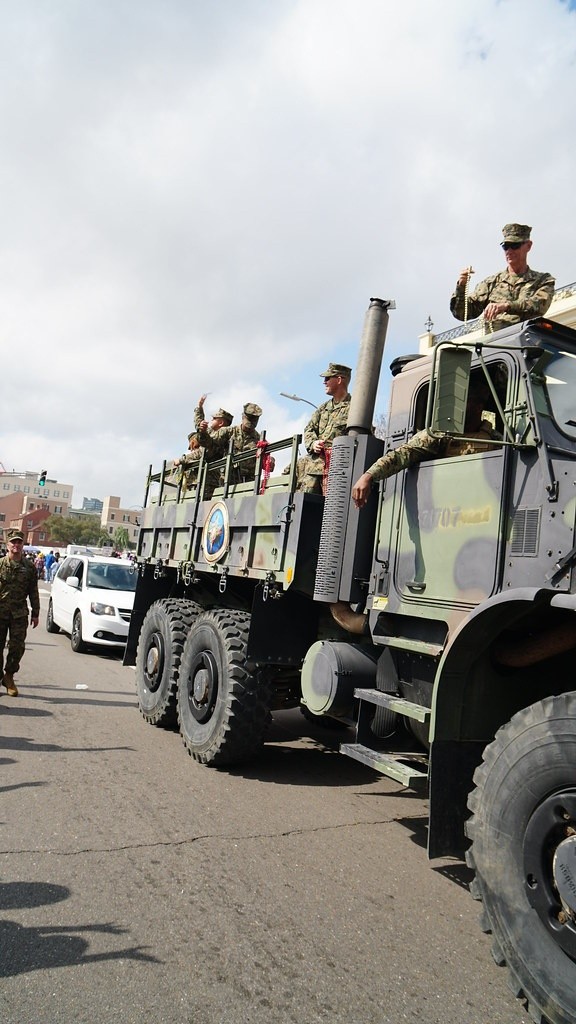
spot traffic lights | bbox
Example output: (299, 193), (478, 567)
(39, 470), (47, 486)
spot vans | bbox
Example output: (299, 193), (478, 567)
(46, 555), (139, 652)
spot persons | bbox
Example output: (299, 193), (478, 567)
(109, 551), (137, 563)
(283, 363), (376, 496)
(169, 393), (234, 501)
(449, 223), (555, 335)
(197, 403), (275, 487)
(0, 531), (40, 696)
(0, 546), (67, 583)
(352, 380), (503, 510)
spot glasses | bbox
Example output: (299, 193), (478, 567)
(501, 241), (528, 251)
(324, 377), (345, 381)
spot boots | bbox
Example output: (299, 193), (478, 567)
(2, 674), (18, 697)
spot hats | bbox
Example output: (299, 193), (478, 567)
(38, 553), (43, 558)
(187, 432), (198, 449)
(467, 381), (491, 401)
(500, 223), (532, 245)
(8, 530), (24, 541)
(319, 363), (352, 379)
(242, 403), (262, 428)
(211, 408), (234, 424)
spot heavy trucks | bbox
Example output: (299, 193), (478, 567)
(123, 298), (576, 1024)
